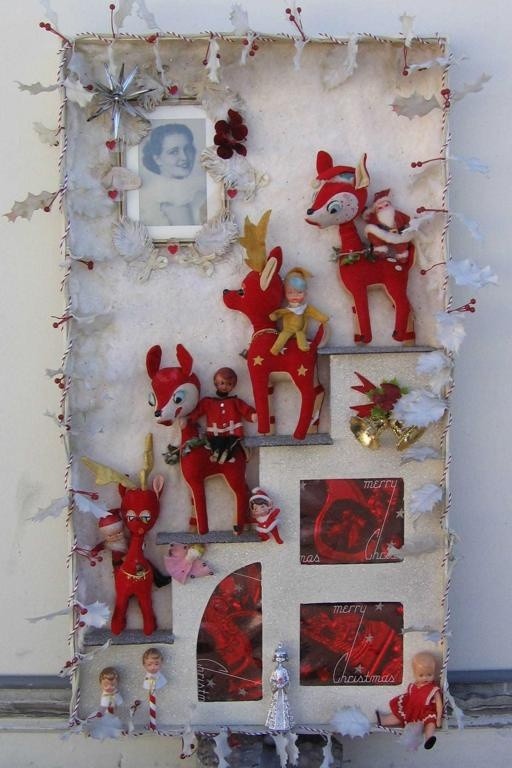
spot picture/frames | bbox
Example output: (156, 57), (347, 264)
(112, 96), (227, 246)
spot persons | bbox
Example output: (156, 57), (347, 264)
(73, 150), (447, 751)
(269, 267), (326, 356)
(139, 123), (199, 226)
(188, 367), (257, 463)
(99, 667), (124, 707)
(264, 643), (295, 730)
(92, 515), (171, 589)
(248, 487), (284, 544)
(376, 652), (444, 751)
(143, 649), (167, 690)
(363, 189), (410, 264)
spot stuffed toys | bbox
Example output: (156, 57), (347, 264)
(146, 344), (253, 537)
(222, 210), (326, 440)
(310, 151), (417, 343)
(80, 433), (165, 637)
